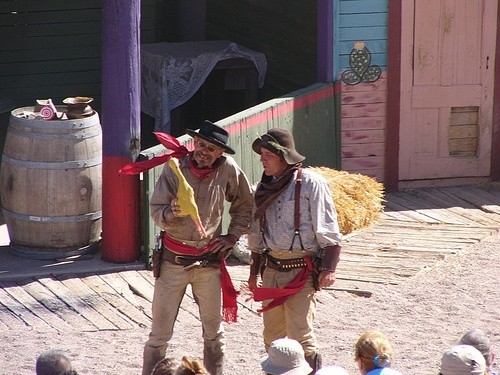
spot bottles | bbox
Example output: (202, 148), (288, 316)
(62, 96), (94, 120)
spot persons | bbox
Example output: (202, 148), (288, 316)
(314, 365), (349, 375)
(118, 121), (253, 375)
(261, 338), (313, 375)
(151, 356), (211, 375)
(354, 329), (403, 375)
(437, 327), (500, 375)
(248, 128), (343, 375)
(36, 349), (80, 375)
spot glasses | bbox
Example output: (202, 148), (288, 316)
(198, 141), (221, 153)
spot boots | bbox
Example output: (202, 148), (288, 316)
(204, 331), (224, 375)
(142, 344), (168, 375)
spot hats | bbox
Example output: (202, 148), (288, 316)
(461, 328), (492, 355)
(185, 120), (236, 155)
(261, 337), (313, 375)
(251, 128), (306, 165)
(439, 344), (486, 375)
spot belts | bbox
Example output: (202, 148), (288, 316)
(160, 246), (218, 272)
(266, 252), (322, 272)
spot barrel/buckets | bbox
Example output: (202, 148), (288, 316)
(1, 105), (104, 260)
